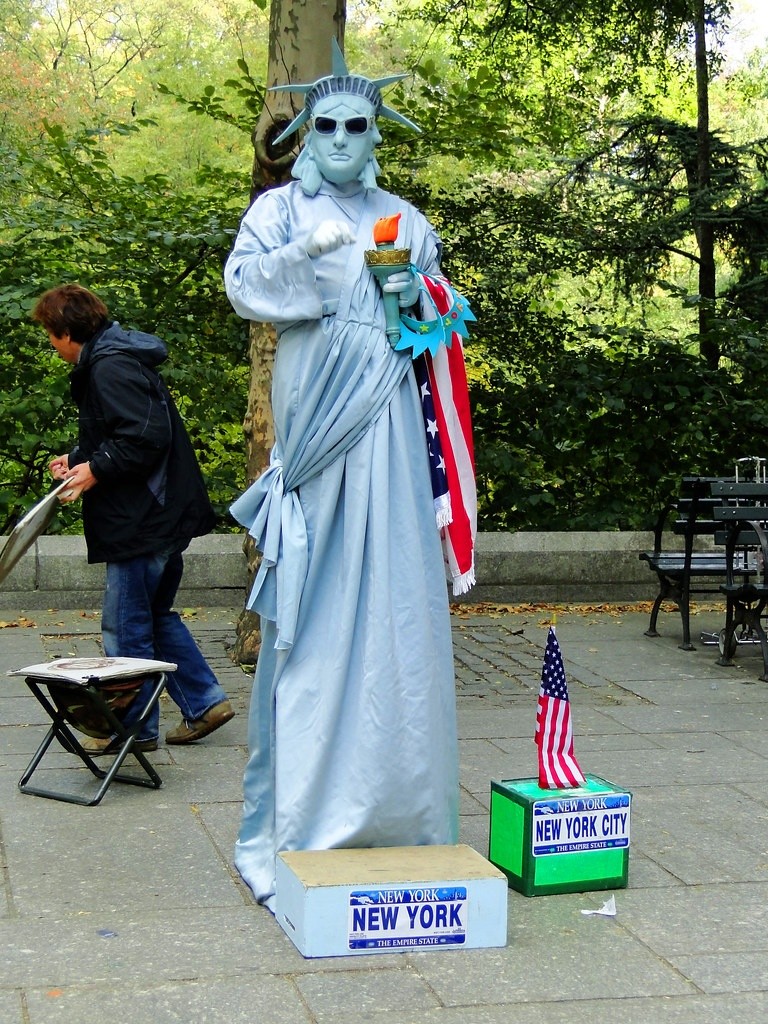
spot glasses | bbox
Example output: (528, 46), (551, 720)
(309, 112), (374, 138)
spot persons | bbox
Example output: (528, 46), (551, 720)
(225, 73), (459, 903)
(36, 285), (235, 754)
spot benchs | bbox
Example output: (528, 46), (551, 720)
(639, 478), (768, 683)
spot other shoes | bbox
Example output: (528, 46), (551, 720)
(77, 733), (158, 756)
(165, 701), (236, 744)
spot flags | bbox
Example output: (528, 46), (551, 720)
(533, 625), (587, 789)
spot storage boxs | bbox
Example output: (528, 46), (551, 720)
(274, 844), (507, 959)
(491, 771), (633, 897)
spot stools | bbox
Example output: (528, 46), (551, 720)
(7, 657), (177, 807)
